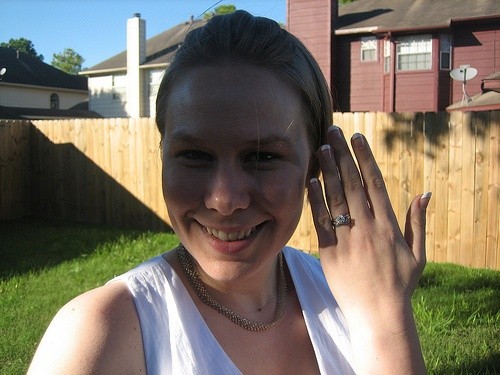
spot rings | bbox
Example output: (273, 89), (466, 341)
(333, 214), (350, 226)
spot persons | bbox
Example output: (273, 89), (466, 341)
(25, 9), (432, 375)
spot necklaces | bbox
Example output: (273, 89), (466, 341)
(178, 243), (287, 331)
